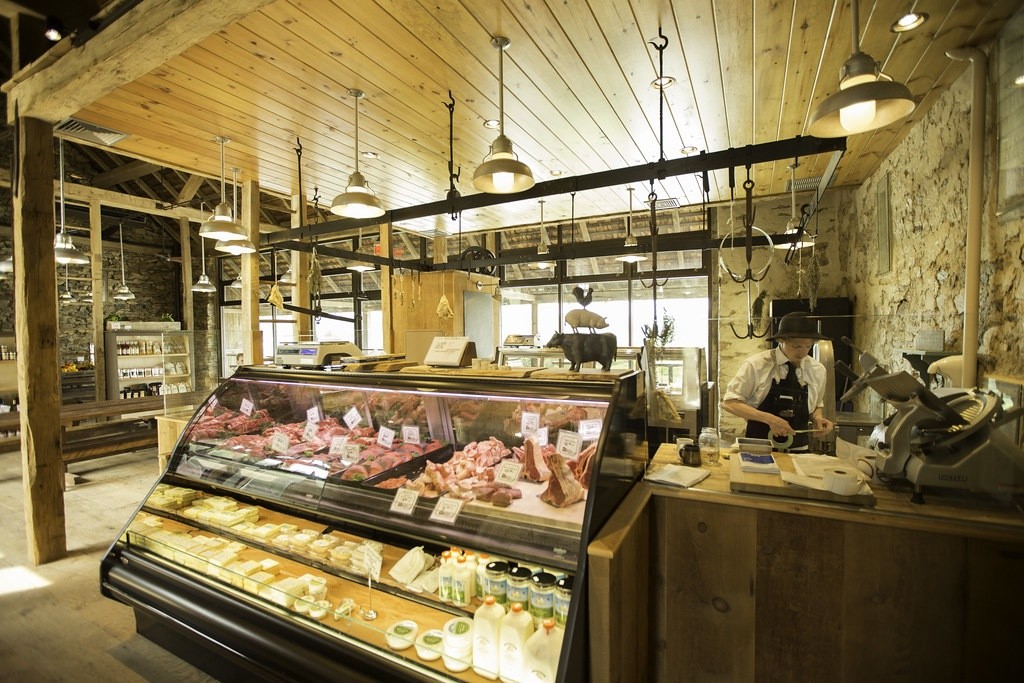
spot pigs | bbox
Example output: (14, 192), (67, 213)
(565, 309), (609, 334)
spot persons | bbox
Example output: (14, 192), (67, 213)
(722, 310), (835, 455)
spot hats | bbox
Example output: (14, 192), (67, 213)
(765, 311), (831, 342)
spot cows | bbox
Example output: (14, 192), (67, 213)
(547, 330), (617, 373)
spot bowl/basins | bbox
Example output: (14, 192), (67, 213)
(383, 615), (474, 673)
(287, 527), (384, 622)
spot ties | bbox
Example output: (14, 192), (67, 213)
(784, 362), (800, 390)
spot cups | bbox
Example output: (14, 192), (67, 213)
(679, 444), (701, 467)
(676, 438), (693, 460)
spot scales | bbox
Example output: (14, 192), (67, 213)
(274, 340), (368, 370)
(503, 334), (543, 348)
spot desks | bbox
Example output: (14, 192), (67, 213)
(59, 398), (208, 486)
(0, 390), (215, 444)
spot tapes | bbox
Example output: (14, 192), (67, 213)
(768, 427), (823, 450)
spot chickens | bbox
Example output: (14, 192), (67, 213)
(572, 287), (594, 309)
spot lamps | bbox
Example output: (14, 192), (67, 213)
(615, 216), (647, 263)
(331, 89), (386, 220)
(537, 200), (549, 254)
(113, 223), (136, 301)
(191, 203), (217, 293)
(214, 167), (255, 255)
(54, 136), (91, 264)
(346, 228), (375, 272)
(471, 37), (535, 195)
(527, 261), (557, 269)
(775, 163), (816, 250)
(624, 187), (639, 246)
(200, 135), (247, 242)
(807, 0), (915, 139)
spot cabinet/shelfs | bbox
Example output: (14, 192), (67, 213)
(104, 321), (194, 420)
(99, 365), (651, 682)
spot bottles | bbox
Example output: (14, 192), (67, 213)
(530, 572), (556, 626)
(506, 567), (533, 614)
(498, 603), (535, 682)
(473, 595), (506, 680)
(553, 578), (575, 630)
(699, 427), (720, 466)
(522, 617), (564, 683)
(116, 339), (169, 356)
(484, 561), (508, 608)
(0, 345), (21, 412)
(439, 546), (491, 607)
